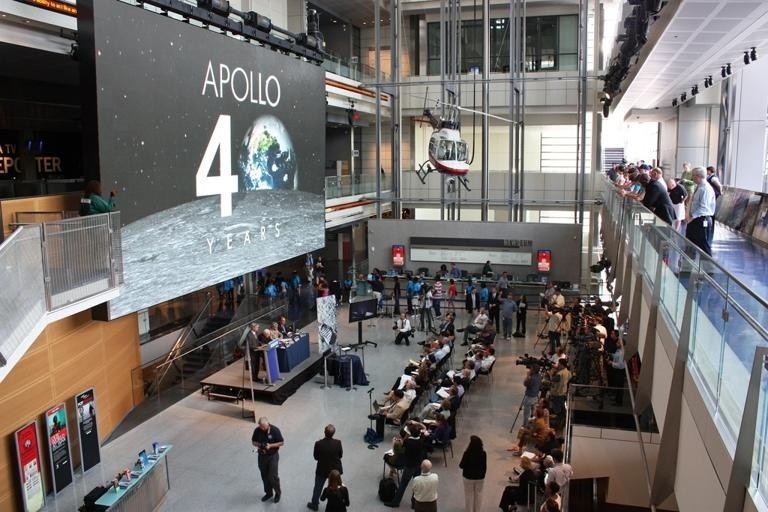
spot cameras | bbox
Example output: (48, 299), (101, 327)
(393, 320), (398, 330)
(259, 443), (266, 454)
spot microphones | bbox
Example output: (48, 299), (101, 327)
(368, 388), (374, 393)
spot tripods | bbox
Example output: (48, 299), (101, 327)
(418, 300), (436, 336)
(542, 319), (577, 354)
(510, 366), (539, 433)
(381, 300), (392, 318)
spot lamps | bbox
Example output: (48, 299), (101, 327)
(593, 0), (667, 119)
(671, 45), (758, 107)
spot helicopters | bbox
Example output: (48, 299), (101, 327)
(410, 85), (520, 191)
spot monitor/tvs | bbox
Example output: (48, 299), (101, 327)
(349, 299), (377, 322)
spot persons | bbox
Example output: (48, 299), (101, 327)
(77, 181), (116, 277)
(607, 159), (723, 276)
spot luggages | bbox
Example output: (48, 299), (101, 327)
(378, 462), (396, 503)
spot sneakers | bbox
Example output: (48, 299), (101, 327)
(307, 502), (319, 511)
(504, 446), (521, 456)
(261, 491), (281, 503)
(508, 467), (522, 483)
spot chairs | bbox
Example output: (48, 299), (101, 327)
(525, 273), (539, 281)
(507, 274), (512, 280)
(417, 267), (429, 276)
(380, 311), (567, 512)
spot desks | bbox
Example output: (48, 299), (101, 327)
(333, 354), (371, 389)
(84, 442), (175, 511)
(273, 330), (311, 373)
